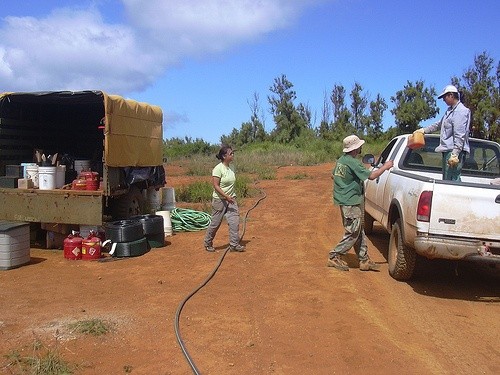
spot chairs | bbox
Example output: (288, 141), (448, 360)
(463, 158), (478, 169)
(407, 153), (424, 164)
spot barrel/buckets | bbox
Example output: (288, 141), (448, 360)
(26, 166), (39, 189)
(56, 164), (67, 189)
(142, 187), (176, 237)
(37, 166), (56, 190)
(74, 160), (91, 176)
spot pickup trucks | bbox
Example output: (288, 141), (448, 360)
(362, 133), (500, 281)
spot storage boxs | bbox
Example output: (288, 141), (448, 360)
(40, 222), (71, 249)
(18, 179), (32, 189)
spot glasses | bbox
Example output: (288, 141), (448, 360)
(224, 151), (232, 155)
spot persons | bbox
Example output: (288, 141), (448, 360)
(328, 134), (394, 270)
(204, 147), (244, 253)
(413, 84), (471, 182)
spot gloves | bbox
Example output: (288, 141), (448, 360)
(413, 128), (424, 135)
(448, 153), (459, 168)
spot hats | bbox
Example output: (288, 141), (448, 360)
(342, 135), (365, 153)
(438, 85), (458, 99)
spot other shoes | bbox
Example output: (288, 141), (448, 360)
(328, 257), (349, 271)
(230, 245), (244, 252)
(360, 259), (380, 270)
(205, 244), (215, 252)
